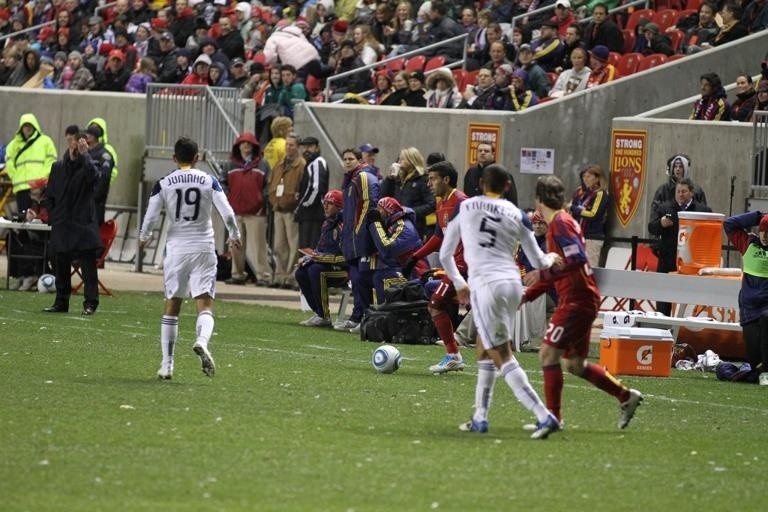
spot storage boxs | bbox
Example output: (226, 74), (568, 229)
(597, 328), (675, 379)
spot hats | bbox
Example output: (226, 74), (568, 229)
(358, 144), (379, 154)
(759, 214), (768, 231)
(324, 190), (344, 208)
(84, 125), (102, 137)
(378, 197), (403, 214)
(299, 136), (319, 146)
(28, 177), (48, 189)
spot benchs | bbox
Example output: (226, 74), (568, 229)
(588, 267), (741, 334)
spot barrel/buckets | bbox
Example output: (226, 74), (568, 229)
(677, 210), (726, 276)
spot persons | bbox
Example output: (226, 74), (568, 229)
(518, 175), (645, 441)
(0, 1), (767, 333)
(438, 164), (568, 441)
(403, 158), (470, 375)
(138, 136), (243, 380)
(722, 209), (768, 384)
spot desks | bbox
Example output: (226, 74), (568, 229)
(0, 220), (52, 289)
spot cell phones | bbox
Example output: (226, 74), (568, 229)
(666, 214), (672, 220)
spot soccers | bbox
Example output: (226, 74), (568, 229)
(372, 344), (401, 374)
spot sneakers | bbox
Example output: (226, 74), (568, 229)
(226, 277), (246, 284)
(522, 417), (565, 431)
(619, 389), (643, 429)
(458, 416), (488, 433)
(19, 275), (38, 291)
(429, 354), (465, 371)
(758, 373), (768, 385)
(531, 414), (559, 440)
(298, 312), (362, 334)
(256, 279), (267, 287)
(192, 341), (216, 376)
(157, 368), (173, 379)
(9, 276), (24, 290)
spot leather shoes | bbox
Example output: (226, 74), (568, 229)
(43, 304), (69, 312)
(82, 307), (94, 315)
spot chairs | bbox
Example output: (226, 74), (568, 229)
(67, 218), (121, 297)
